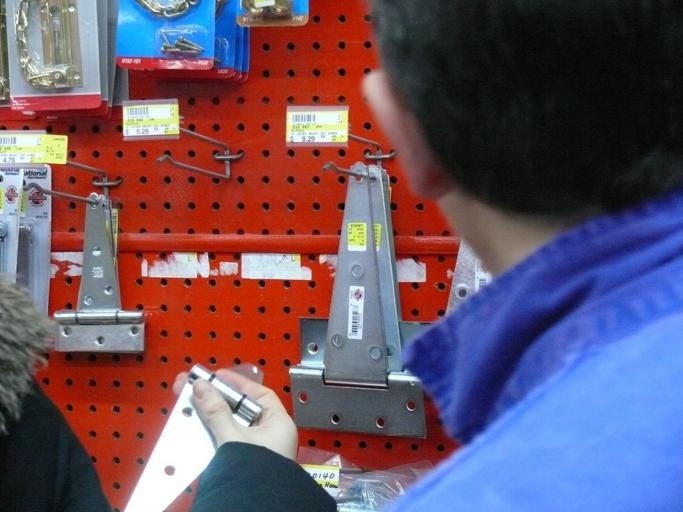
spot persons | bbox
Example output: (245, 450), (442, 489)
(359, 1), (683, 512)
(0, 274), (338, 512)
